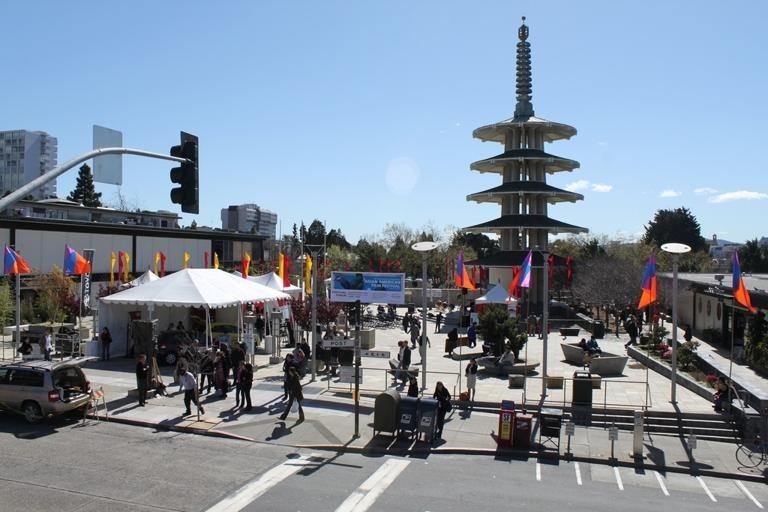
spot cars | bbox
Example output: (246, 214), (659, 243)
(153, 328), (233, 365)
(190, 315), (261, 353)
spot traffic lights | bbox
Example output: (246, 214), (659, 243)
(170, 130), (200, 214)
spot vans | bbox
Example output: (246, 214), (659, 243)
(0, 355), (99, 425)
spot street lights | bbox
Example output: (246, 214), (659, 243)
(658, 240), (693, 407)
(303, 244), (325, 382)
(410, 240), (438, 390)
(532, 243), (560, 400)
(296, 254), (306, 288)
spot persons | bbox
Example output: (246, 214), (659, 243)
(712, 377), (728, 413)
(528, 313), (544, 337)
(377, 303), (479, 439)
(101, 327), (112, 362)
(684, 325), (692, 341)
(572, 303), (672, 357)
(136, 302), (267, 417)
(497, 347), (515, 375)
(316, 326), (354, 377)
(276, 337), (311, 423)
(20, 329), (55, 361)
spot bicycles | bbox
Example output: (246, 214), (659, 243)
(734, 431), (767, 469)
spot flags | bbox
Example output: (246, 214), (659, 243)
(5, 244), (32, 274)
(637, 248), (657, 309)
(730, 249), (757, 314)
(110, 249), (312, 293)
(456, 248), (571, 291)
(64, 244), (91, 275)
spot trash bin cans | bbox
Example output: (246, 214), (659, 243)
(416, 398), (439, 445)
(374, 389), (401, 440)
(397, 397), (418, 442)
(515, 413), (533, 449)
(408, 303), (415, 313)
(592, 319), (604, 339)
(572, 370), (592, 407)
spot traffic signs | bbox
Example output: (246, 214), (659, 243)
(323, 339), (354, 348)
(360, 350), (389, 358)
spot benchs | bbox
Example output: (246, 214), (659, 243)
(560, 341), (628, 375)
(479, 359), (539, 375)
